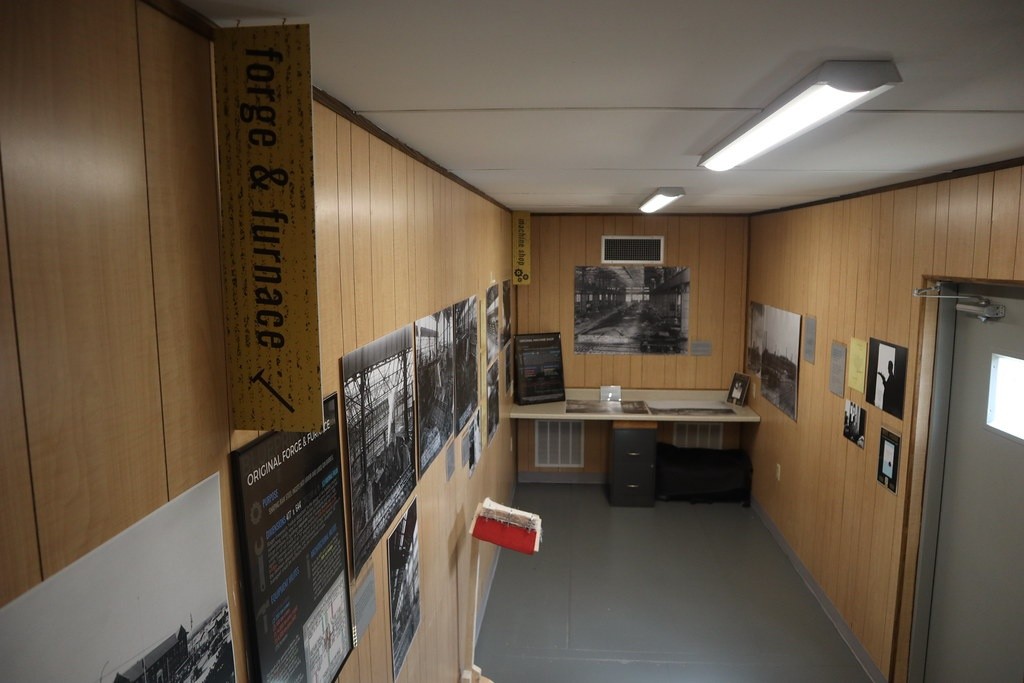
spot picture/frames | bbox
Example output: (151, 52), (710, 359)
(227, 390), (355, 683)
(513, 332), (566, 407)
(725, 371), (752, 409)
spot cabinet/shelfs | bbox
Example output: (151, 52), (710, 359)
(606, 420), (658, 508)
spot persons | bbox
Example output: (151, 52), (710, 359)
(878, 360), (894, 414)
(373, 436), (411, 502)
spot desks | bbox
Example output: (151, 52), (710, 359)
(510, 387), (760, 423)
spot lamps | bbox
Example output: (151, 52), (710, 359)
(637, 187), (686, 213)
(699, 61), (902, 172)
